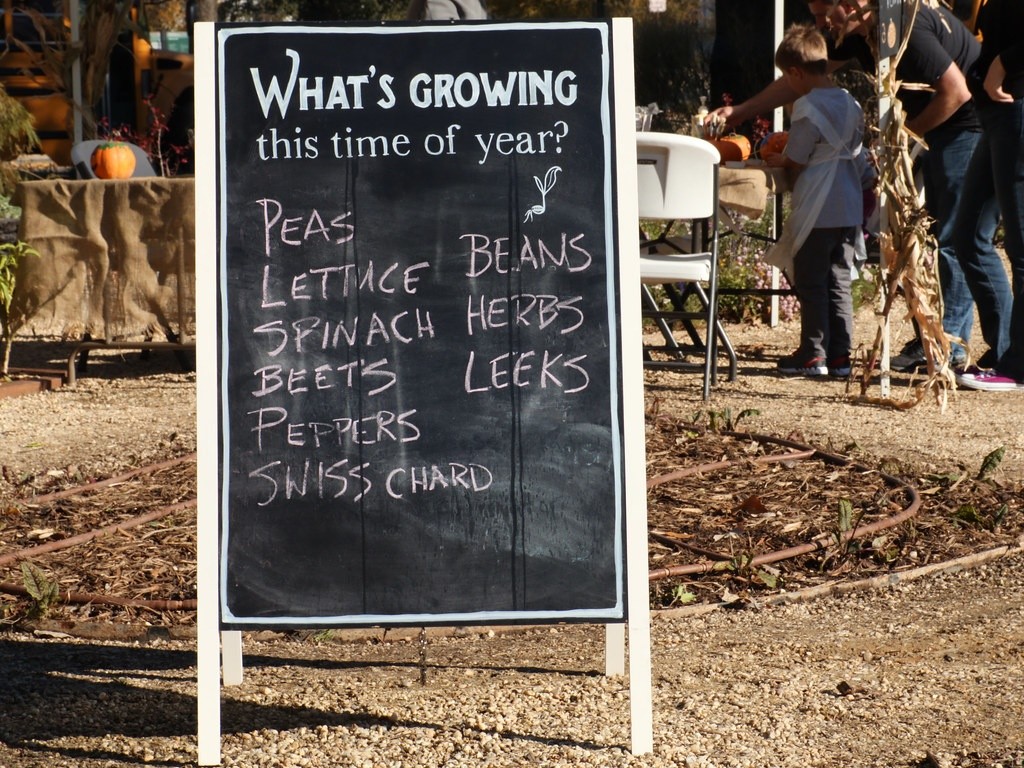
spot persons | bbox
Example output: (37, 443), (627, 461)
(703, 0), (1024, 393)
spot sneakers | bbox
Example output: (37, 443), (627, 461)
(953, 364), (1022, 392)
(777, 350), (827, 374)
(884, 337), (928, 370)
(828, 356), (853, 377)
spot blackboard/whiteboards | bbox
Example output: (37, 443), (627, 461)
(192, 16), (649, 627)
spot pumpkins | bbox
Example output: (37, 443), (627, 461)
(89, 139), (137, 180)
(706, 131), (793, 163)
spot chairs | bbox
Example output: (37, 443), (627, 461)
(71, 140), (193, 371)
(636, 130), (737, 403)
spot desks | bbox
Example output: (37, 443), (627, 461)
(712, 158), (801, 330)
(15, 174), (196, 391)
